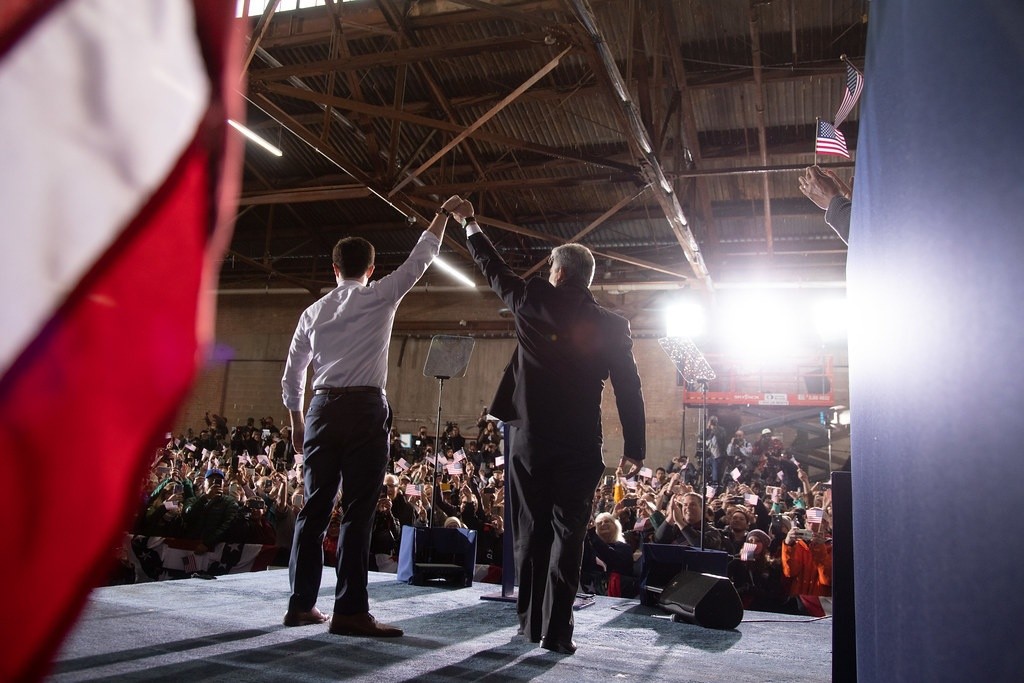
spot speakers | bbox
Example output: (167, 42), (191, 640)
(655, 569), (744, 630)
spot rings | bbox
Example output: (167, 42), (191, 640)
(804, 183), (807, 188)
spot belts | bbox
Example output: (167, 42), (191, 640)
(316, 386), (386, 396)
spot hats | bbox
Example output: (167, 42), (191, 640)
(205, 469), (224, 479)
(747, 530), (772, 549)
(762, 429), (771, 435)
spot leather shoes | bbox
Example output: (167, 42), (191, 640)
(283, 607), (331, 627)
(518, 627), (542, 643)
(540, 638), (576, 654)
(329, 612), (404, 638)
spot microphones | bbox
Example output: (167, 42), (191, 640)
(649, 613), (682, 624)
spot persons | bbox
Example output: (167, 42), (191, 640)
(280, 194), (464, 638)
(137, 410), (831, 615)
(452, 195), (647, 653)
(795, 164), (854, 246)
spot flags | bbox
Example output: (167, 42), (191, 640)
(120, 533), (281, 585)
(834, 63), (865, 127)
(816, 120), (851, 158)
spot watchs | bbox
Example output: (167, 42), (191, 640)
(462, 216), (475, 229)
(435, 207), (449, 217)
